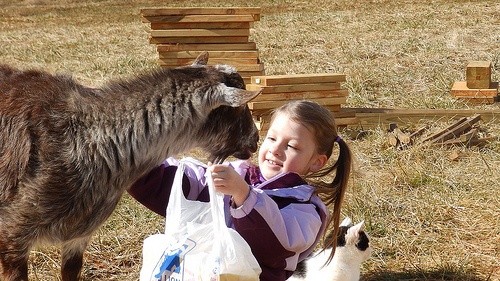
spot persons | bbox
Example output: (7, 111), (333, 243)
(126, 100), (356, 281)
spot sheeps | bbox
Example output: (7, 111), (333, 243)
(0, 50), (266, 281)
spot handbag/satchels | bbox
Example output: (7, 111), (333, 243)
(139, 157), (262, 281)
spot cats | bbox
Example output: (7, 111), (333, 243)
(284, 216), (373, 281)
